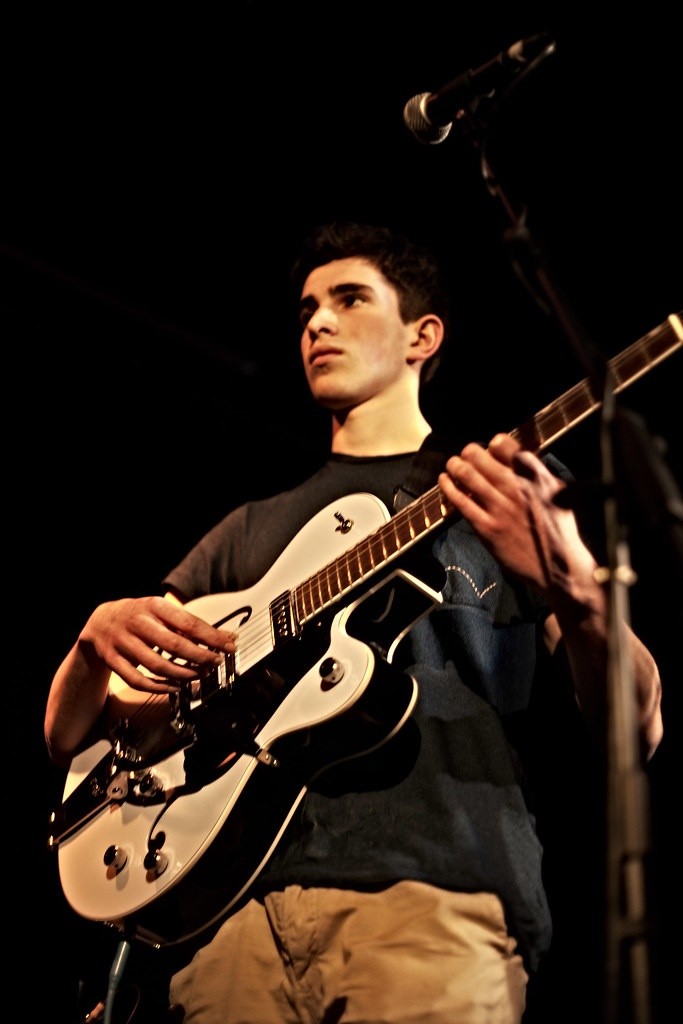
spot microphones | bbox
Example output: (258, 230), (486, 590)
(404, 32), (555, 145)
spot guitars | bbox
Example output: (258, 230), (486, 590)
(46, 311), (683, 952)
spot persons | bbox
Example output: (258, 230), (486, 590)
(43, 224), (664, 1024)
